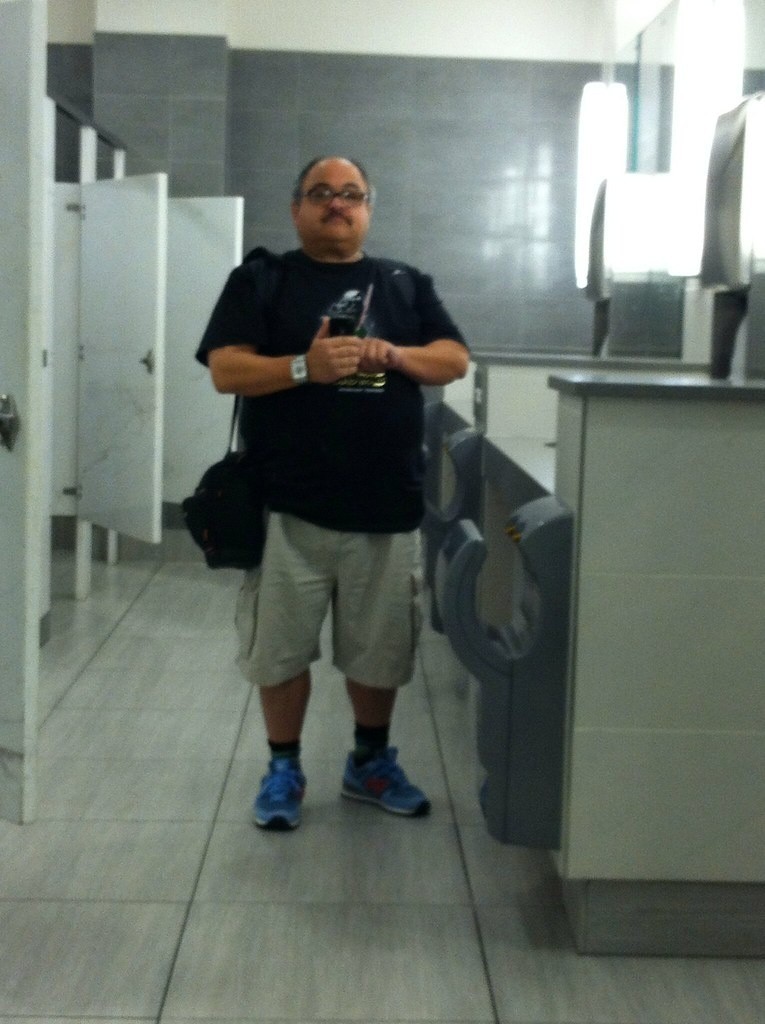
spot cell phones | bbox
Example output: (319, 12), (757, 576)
(329, 316), (356, 338)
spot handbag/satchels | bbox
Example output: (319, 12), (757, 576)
(181, 452), (268, 568)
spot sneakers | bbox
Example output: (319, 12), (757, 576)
(252, 757), (306, 828)
(341, 747), (432, 815)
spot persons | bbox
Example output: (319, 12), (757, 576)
(193, 154), (473, 831)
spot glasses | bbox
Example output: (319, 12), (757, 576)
(302, 184), (368, 207)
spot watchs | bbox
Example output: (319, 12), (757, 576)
(291, 354), (310, 385)
